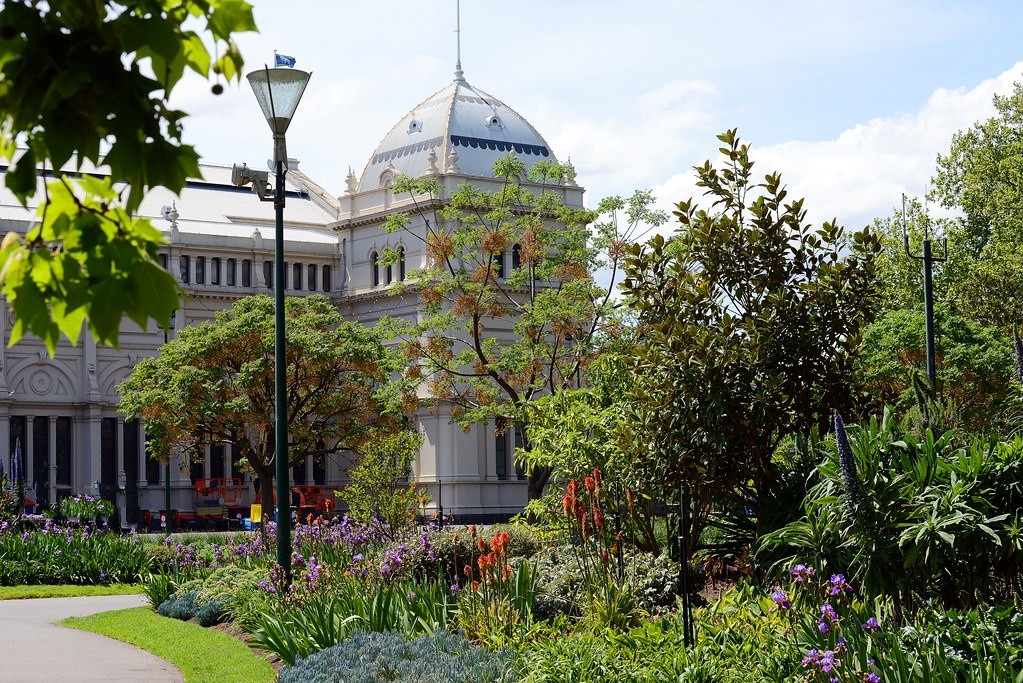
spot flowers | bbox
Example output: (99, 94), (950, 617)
(50, 495), (114, 525)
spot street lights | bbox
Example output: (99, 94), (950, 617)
(231, 66), (314, 602)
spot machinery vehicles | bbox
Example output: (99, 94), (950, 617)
(177, 478), (347, 532)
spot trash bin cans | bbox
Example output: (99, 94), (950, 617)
(289, 506), (297, 528)
(300, 505), (315, 525)
(274, 505), (277, 524)
(158, 509), (177, 528)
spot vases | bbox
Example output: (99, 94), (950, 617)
(67, 516), (80, 523)
(98, 515), (108, 524)
